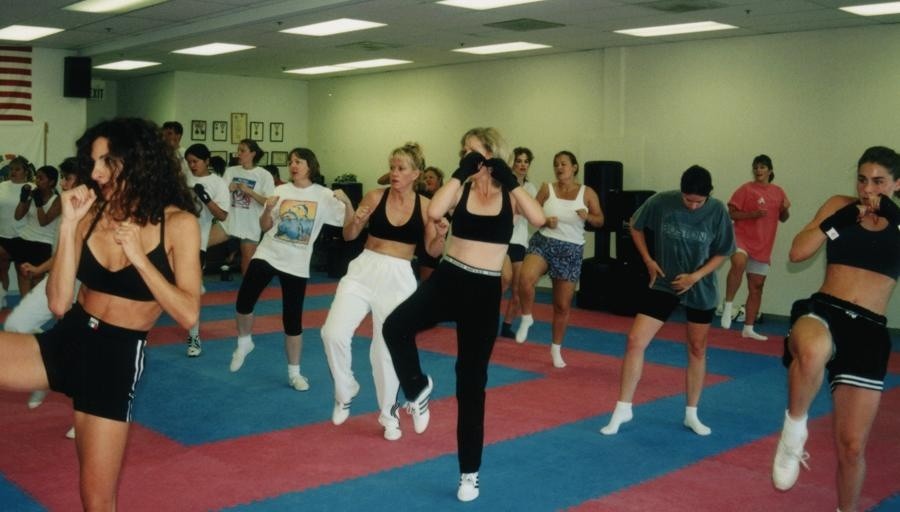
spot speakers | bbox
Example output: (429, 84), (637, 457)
(584, 160), (624, 232)
(63, 56), (92, 98)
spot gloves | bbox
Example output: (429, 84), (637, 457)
(451, 151), (487, 187)
(20, 184), (31, 201)
(194, 183), (211, 205)
(31, 188), (42, 208)
(486, 156), (521, 192)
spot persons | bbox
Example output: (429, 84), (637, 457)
(769, 145), (900, 512)
(183, 142), (231, 356)
(0, 156), (107, 439)
(515, 151), (605, 369)
(0, 117), (203, 512)
(599, 165), (736, 435)
(412, 166), (445, 291)
(206, 137), (274, 275)
(164, 121), (190, 173)
(377, 172), (391, 185)
(230, 148), (354, 390)
(498, 146), (538, 337)
(719, 154), (791, 341)
(381, 125), (547, 501)
(320, 139), (450, 440)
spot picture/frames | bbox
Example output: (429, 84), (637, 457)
(190, 112), (289, 171)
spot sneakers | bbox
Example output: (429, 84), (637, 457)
(288, 374), (310, 391)
(378, 407), (403, 441)
(230, 342), (254, 372)
(332, 383), (360, 426)
(736, 305), (760, 323)
(457, 471), (481, 502)
(773, 410), (810, 490)
(715, 305), (738, 320)
(402, 375), (434, 434)
(187, 334), (203, 357)
(221, 270), (233, 282)
